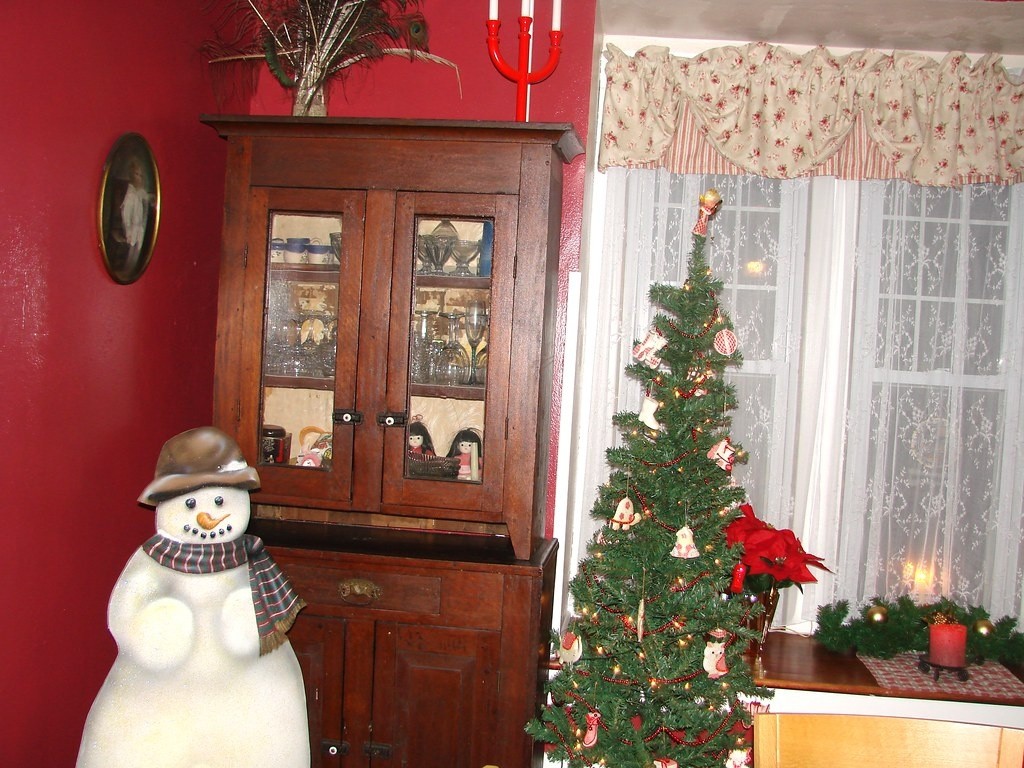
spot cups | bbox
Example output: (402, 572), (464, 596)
(286, 237), (310, 245)
(270, 243), (286, 263)
(308, 245), (334, 265)
(283, 244), (309, 264)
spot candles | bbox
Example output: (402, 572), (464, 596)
(552, 0), (562, 31)
(929, 623), (967, 667)
(489, 0), (499, 20)
(521, 0), (529, 17)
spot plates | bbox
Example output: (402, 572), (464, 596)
(408, 449), (462, 480)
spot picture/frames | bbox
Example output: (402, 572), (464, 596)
(98, 132), (162, 285)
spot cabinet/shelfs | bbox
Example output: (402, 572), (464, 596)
(200, 113), (586, 559)
(746, 632), (1024, 768)
(245, 519), (560, 768)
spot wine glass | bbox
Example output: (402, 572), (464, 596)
(414, 219), (483, 276)
(263, 296), (491, 384)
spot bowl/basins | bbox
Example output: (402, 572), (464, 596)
(329, 232), (342, 264)
